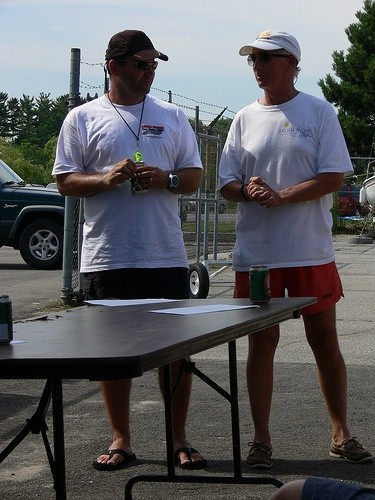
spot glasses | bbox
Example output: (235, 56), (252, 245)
(132, 59), (159, 71)
(246, 51), (293, 67)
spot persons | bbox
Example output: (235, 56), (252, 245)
(215, 30), (373, 471)
(52, 29), (211, 468)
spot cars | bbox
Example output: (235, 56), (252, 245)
(182, 192), (226, 215)
(46, 183), (58, 190)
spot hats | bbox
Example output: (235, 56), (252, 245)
(239, 30), (301, 64)
(105, 29), (169, 63)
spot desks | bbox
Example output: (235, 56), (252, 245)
(0, 297), (318, 500)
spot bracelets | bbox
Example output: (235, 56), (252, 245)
(240, 185), (251, 201)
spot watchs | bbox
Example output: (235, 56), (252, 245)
(166, 170), (179, 190)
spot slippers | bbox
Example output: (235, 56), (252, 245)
(92, 449), (137, 471)
(174, 447), (207, 470)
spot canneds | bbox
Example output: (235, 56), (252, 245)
(130, 161), (148, 195)
(0, 295), (13, 345)
(249, 265), (271, 303)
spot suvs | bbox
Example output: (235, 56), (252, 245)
(0, 159), (65, 271)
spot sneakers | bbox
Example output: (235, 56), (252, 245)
(243, 440), (275, 469)
(329, 435), (375, 464)
